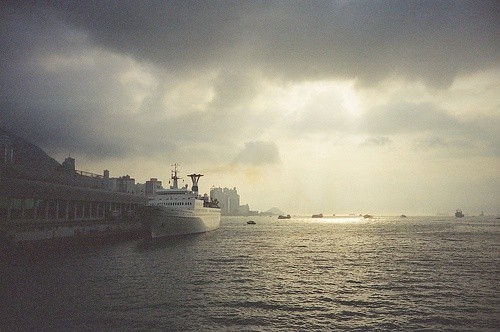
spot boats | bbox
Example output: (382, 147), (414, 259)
(455, 209), (464, 217)
(278, 215), (291, 219)
(400, 215), (406, 217)
(312, 214), (323, 218)
(363, 214), (372, 219)
(135, 163), (222, 241)
(247, 221), (257, 224)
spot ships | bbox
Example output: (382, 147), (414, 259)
(1, 134), (149, 246)
